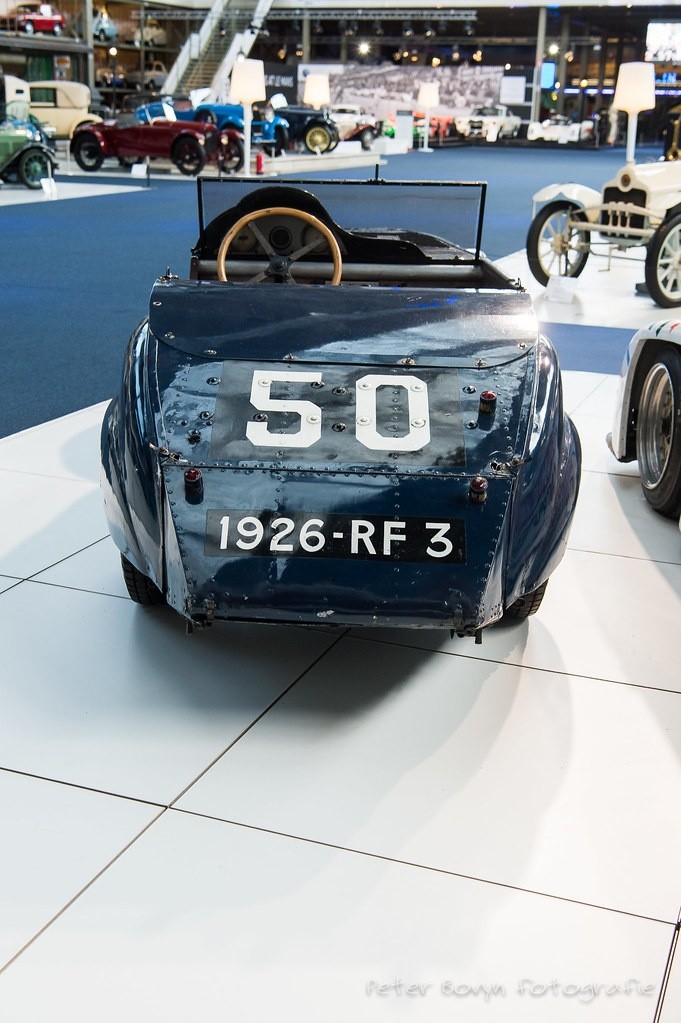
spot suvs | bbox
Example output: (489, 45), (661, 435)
(459, 104), (521, 143)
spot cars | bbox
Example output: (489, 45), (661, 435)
(263, 108), (340, 154)
(129, 93), (289, 158)
(320, 103), (382, 150)
(71, 6), (118, 41)
(69, 94), (246, 174)
(124, 20), (167, 47)
(100, 161), (583, 645)
(126, 60), (169, 92)
(525, 158), (681, 309)
(0, 101), (60, 189)
(604, 319), (681, 521)
(528, 118), (594, 145)
(0, 3), (65, 36)
(30, 80), (100, 141)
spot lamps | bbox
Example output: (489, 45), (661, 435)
(302, 72), (332, 111)
(415, 81), (440, 153)
(228, 59), (266, 176)
(613, 62), (657, 161)
